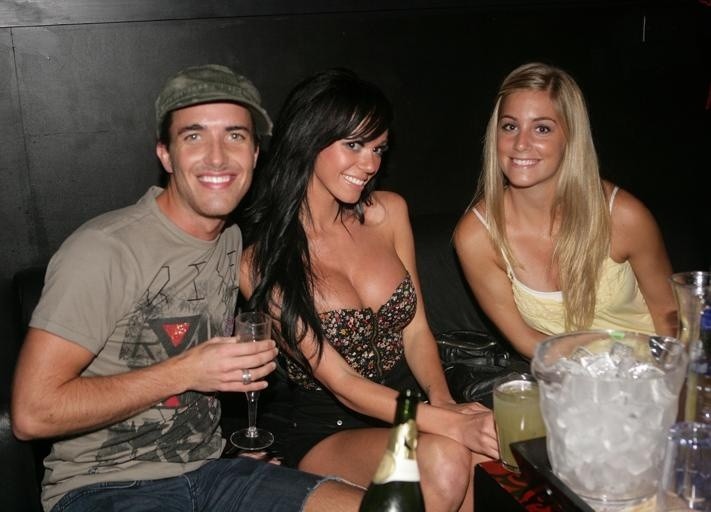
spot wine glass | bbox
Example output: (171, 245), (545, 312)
(231, 312), (274, 450)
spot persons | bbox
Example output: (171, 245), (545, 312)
(453, 62), (700, 426)
(11, 63), (365, 512)
(240, 71), (501, 511)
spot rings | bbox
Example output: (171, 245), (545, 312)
(242, 369), (251, 385)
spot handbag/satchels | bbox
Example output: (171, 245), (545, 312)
(435, 330), (532, 402)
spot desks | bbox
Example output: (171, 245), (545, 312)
(472, 458), (580, 512)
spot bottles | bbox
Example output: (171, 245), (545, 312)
(353, 386), (425, 512)
(662, 271), (710, 512)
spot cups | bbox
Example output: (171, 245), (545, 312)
(532, 330), (689, 507)
(493, 374), (546, 472)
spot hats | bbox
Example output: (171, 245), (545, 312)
(155, 63), (273, 139)
